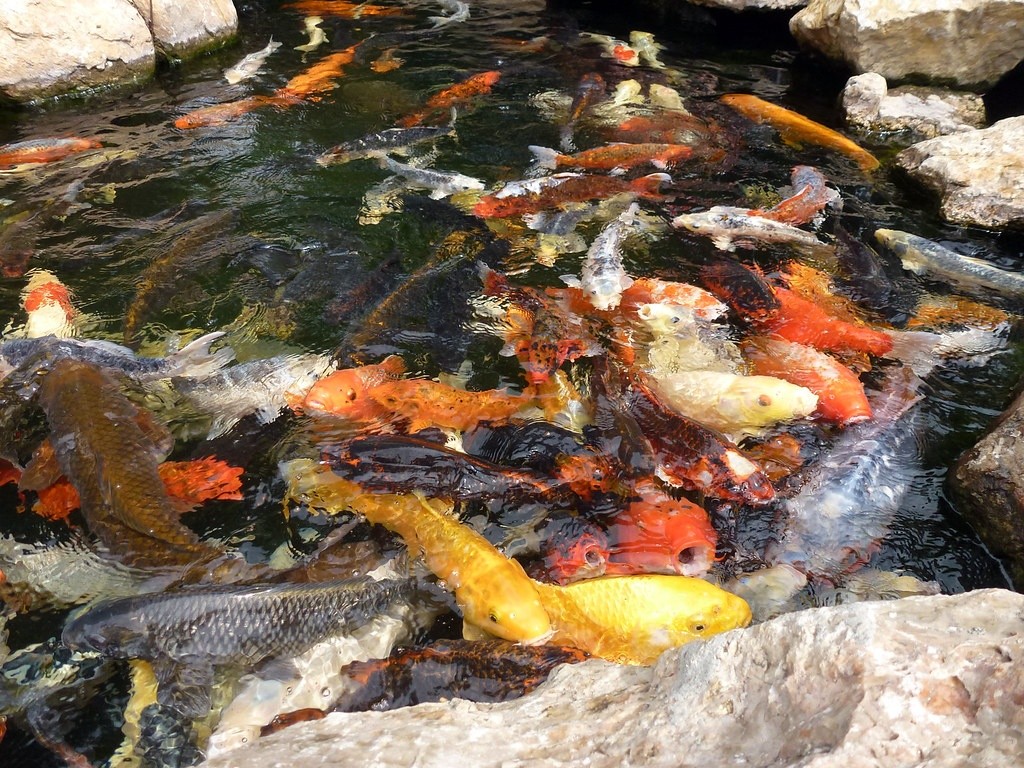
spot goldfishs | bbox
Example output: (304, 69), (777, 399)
(0, 1), (1023, 767)
